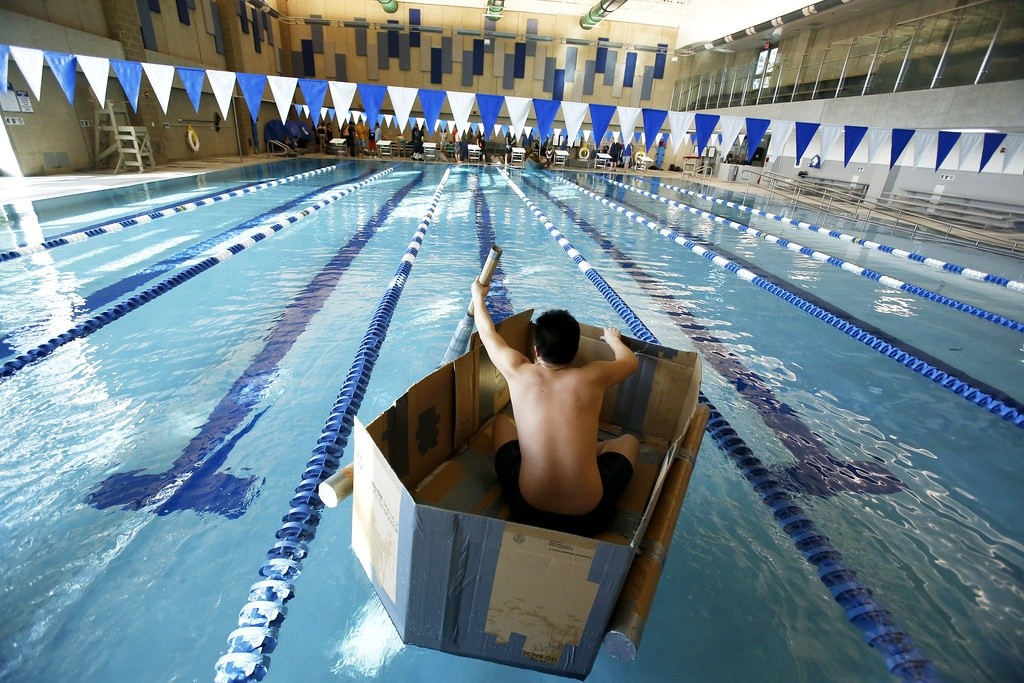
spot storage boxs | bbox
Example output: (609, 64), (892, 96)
(351, 306), (703, 682)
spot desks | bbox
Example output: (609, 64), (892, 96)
(718, 163), (764, 183)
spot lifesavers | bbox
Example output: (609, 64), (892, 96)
(579, 147), (590, 160)
(635, 151), (646, 164)
(187, 129), (200, 152)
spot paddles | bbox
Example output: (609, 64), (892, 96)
(445, 244), (503, 367)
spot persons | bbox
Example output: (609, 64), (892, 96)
(471, 276), (641, 538)
(313, 121), (635, 173)
